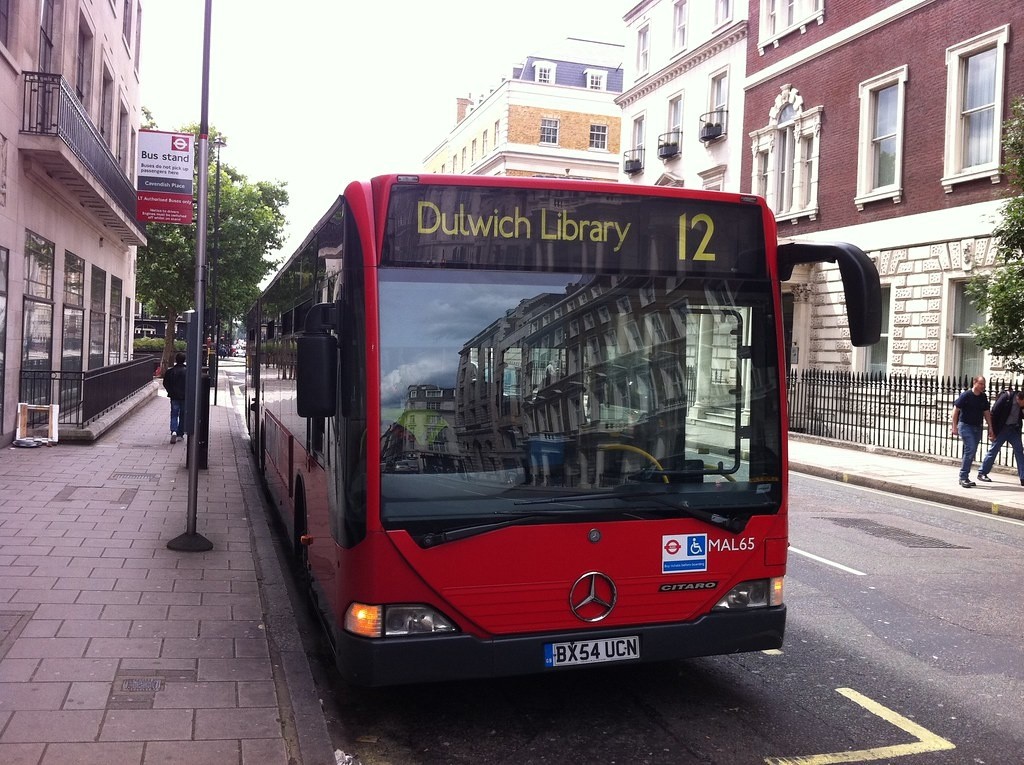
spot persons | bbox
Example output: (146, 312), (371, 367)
(952, 374), (1024, 488)
(163, 352), (186, 443)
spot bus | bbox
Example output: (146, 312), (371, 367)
(241, 172), (883, 690)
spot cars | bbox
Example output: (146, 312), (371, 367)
(230, 339), (246, 357)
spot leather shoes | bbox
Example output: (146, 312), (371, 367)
(977, 474), (991, 482)
(968, 478), (976, 486)
(959, 479), (970, 488)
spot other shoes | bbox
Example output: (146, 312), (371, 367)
(170, 432), (177, 443)
(177, 437), (184, 440)
